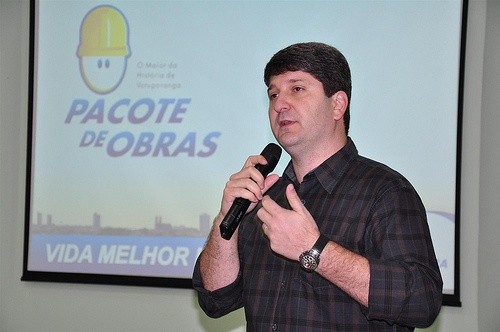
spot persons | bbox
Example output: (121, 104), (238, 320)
(191, 42), (443, 332)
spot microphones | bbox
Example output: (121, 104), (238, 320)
(219, 143), (282, 241)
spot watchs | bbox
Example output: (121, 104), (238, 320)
(299, 234), (330, 273)
